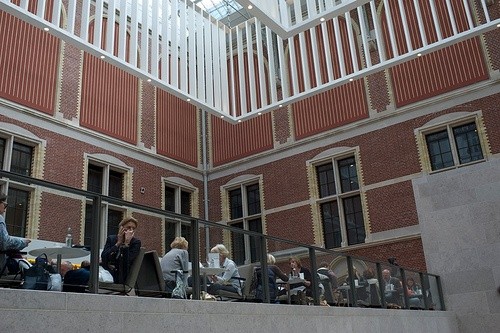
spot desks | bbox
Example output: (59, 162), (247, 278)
(276, 276), (304, 303)
(188, 268), (228, 292)
(338, 286), (350, 307)
(28, 247), (90, 274)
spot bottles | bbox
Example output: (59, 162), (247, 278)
(66, 234), (72, 248)
(284, 272), (292, 279)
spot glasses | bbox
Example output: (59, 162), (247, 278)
(0, 200), (8, 209)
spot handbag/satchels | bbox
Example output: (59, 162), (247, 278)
(99, 266), (114, 283)
(190, 291), (222, 301)
(24, 265), (52, 291)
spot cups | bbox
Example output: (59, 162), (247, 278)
(300, 273), (304, 279)
(354, 279), (358, 286)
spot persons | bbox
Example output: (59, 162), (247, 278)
(342, 265), (369, 300)
(206, 244), (244, 294)
(160, 236), (192, 291)
(317, 263), (344, 304)
(408, 273), (429, 307)
(0, 192), (31, 279)
(279, 256), (312, 305)
(256, 254), (289, 303)
(382, 269), (402, 302)
(362, 267), (381, 303)
(62, 217), (141, 293)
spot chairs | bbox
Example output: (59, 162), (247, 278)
(0, 238), (27, 289)
(138, 251), (193, 299)
(323, 281), (338, 304)
(215, 263), (255, 301)
(88, 246), (146, 295)
